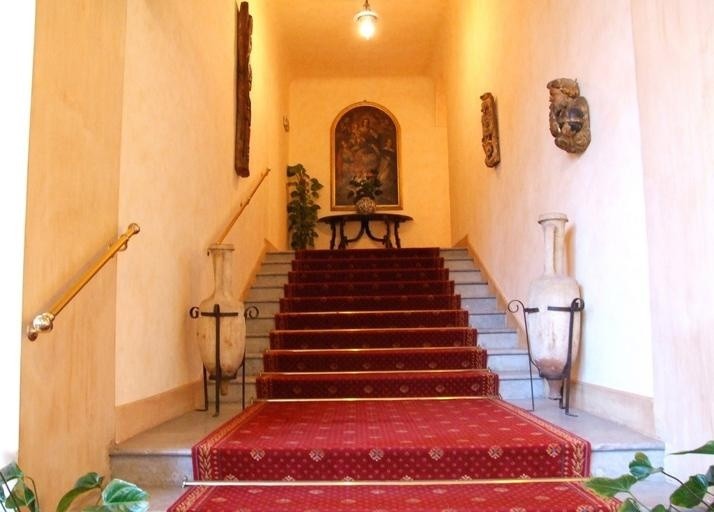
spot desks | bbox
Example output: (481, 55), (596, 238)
(316, 213), (414, 250)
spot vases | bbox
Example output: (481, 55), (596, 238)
(525, 211), (583, 400)
(196, 242), (248, 399)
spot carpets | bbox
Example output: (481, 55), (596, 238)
(167, 247), (621, 512)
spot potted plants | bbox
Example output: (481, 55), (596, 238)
(349, 169), (383, 214)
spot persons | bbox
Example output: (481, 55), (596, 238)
(546, 78), (579, 114)
(341, 118), (394, 183)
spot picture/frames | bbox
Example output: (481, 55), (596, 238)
(329, 99), (403, 211)
